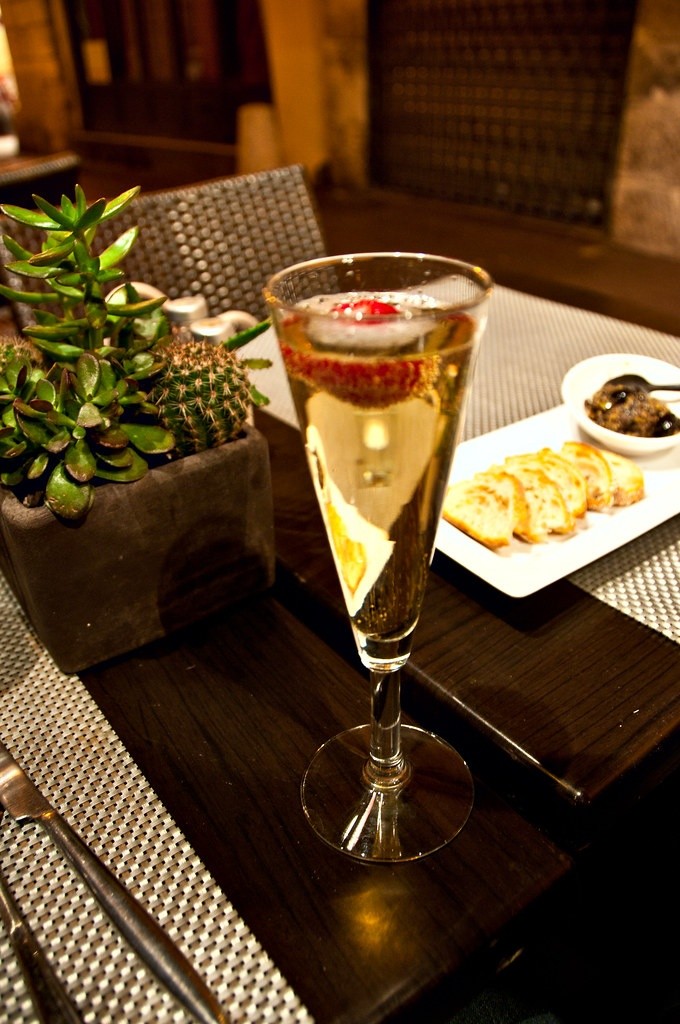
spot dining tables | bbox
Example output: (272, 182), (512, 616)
(2, 271), (679, 1021)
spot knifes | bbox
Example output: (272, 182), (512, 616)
(0, 743), (227, 1023)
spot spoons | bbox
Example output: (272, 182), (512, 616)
(602, 374), (680, 394)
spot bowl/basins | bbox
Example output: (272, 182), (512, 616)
(561, 353), (680, 456)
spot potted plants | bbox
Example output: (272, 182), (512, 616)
(0, 183), (276, 675)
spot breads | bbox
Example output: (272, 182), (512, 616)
(430, 442), (646, 550)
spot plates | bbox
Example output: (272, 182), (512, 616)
(434, 403), (680, 598)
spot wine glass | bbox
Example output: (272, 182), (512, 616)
(265, 251), (493, 861)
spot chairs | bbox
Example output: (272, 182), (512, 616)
(0, 165), (341, 338)
